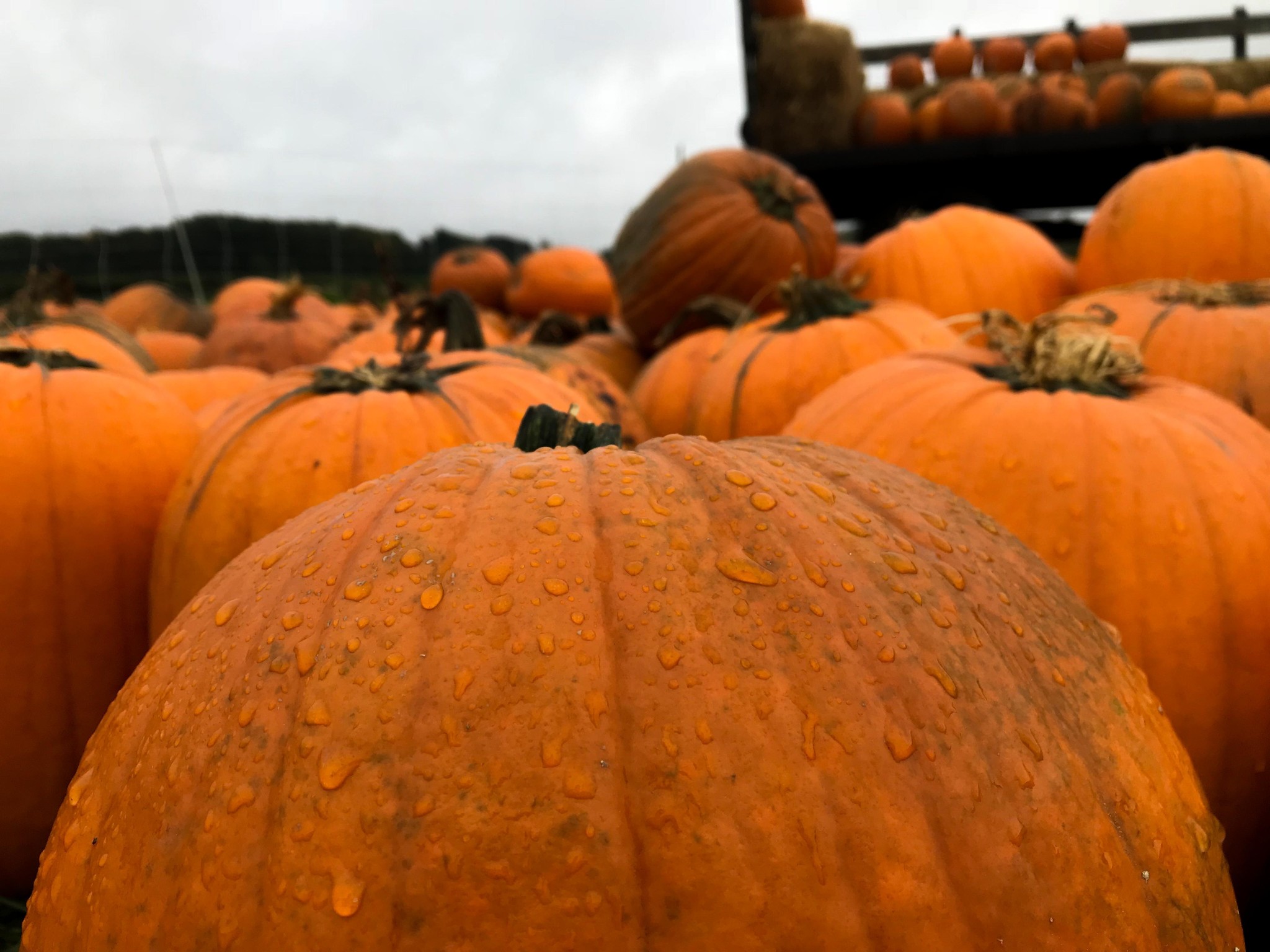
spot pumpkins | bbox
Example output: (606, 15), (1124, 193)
(0, 150), (1270, 952)
(857, 26), (1270, 141)
